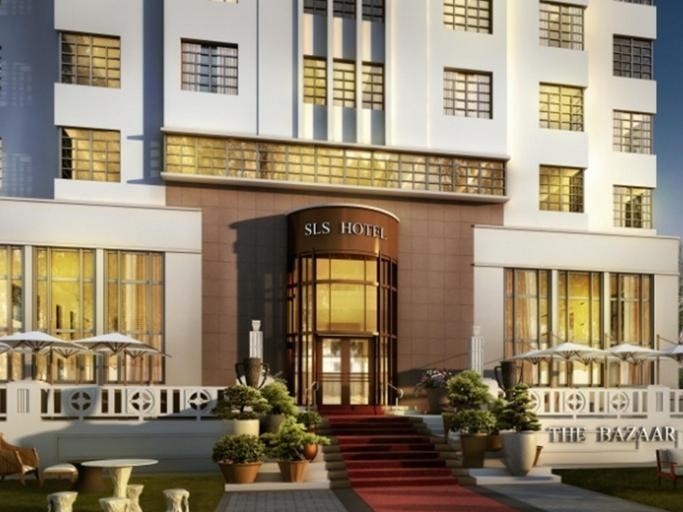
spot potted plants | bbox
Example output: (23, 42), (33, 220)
(414, 367), (542, 477)
(208, 379), (331, 484)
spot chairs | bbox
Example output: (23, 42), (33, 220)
(0, 431), (40, 485)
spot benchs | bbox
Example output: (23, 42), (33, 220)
(655, 447), (683, 492)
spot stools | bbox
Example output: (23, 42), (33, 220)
(47, 481), (191, 512)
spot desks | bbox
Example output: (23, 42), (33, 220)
(80, 457), (159, 497)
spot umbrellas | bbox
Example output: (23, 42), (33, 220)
(504, 346), (561, 390)
(0, 329), (92, 385)
(73, 331), (171, 383)
(574, 339), (683, 389)
(533, 343), (604, 390)
